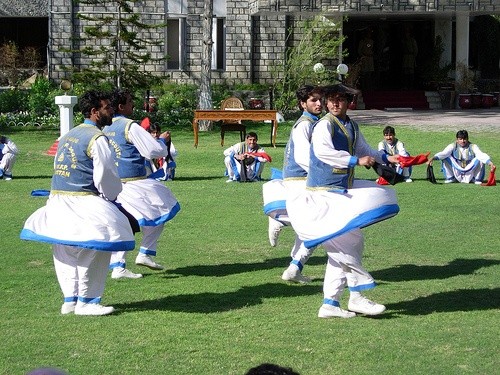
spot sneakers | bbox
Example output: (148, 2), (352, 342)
(282, 269), (311, 283)
(268, 215), (283, 247)
(318, 306), (356, 319)
(4, 175), (12, 180)
(375, 178), (379, 182)
(475, 180), (482, 184)
(404, 177), (413, 183)
(112, 268), (143, 279)
(445, 178), (456, 183)
(347, 297), (386, 315)
(135, 255), (163, 270)
(75, 302), (115, 316)
(61, 303), (76, 315)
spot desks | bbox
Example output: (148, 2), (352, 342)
(193, 109), (278, 149)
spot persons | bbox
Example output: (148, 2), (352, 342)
(243, 363), (298, 375)
(283, 84), (403, 320)
(223, 131), (271, 182)
(143, 124), (177, 181)
(0, 135), (18, 182)
(102, 88), (181, 279)
(22, 89), (135, 317)
(263, 85), (323, 284)
(427, 130), (496, 185)
(377, 125), (413, 184)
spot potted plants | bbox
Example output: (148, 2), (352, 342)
(424, 82), (442, 109)
(470, 81), (481, 105)
(493, 80), (500, 107)
(455, 79), (476, 110)
(438, 77), (456, 110)
(481, 80), (495, 107)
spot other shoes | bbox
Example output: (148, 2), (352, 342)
(253, 178), (258, 182)
(226, 179), (233, 182)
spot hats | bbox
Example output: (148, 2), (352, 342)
(313, 84), (360, 96)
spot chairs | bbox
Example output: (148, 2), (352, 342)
(220, 97), (246, 147)
